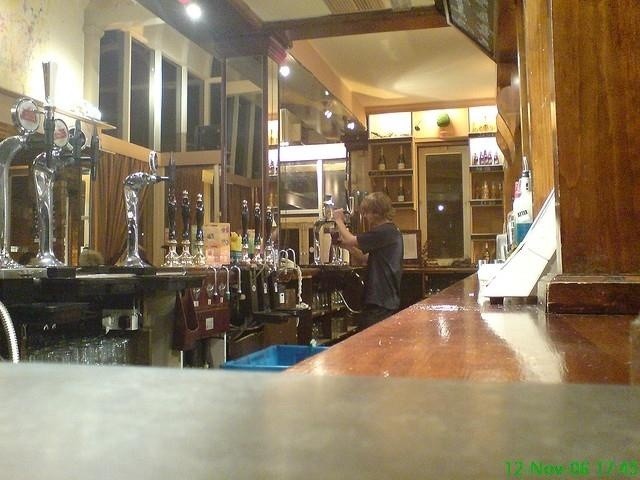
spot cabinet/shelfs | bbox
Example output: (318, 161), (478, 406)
(367, 137), (416, 209)
(463, 132), (505, 241)
(293, 265), (364, 349)
(367, 105), (502, 146)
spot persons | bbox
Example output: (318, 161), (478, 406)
(332, 190), (406, 330)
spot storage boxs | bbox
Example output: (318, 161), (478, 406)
(218, 343), (333, 372)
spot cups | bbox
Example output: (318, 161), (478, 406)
(25, 331), (132, 364)
(496, 234), (508, 264)
(477, 259), (487, 270)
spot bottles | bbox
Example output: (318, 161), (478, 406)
(475, 181), (504, 200)
(472, 112), (497, 131)
(383, 177), (405, 203)
(472, 149), (500, 165)
(377, 145), (406, 170)
(482, 242), (491, 264)
(506, 168), (532, 252)
